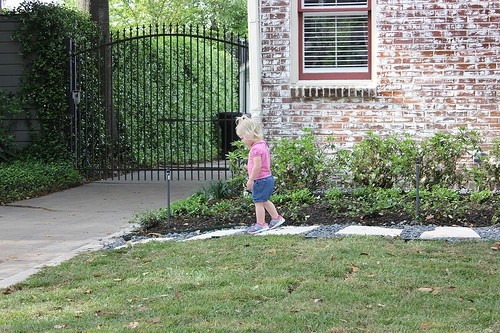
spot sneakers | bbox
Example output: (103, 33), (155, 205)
(269, 216), (285, 229)
(248, 221), (269, 233)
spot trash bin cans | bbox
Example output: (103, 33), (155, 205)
(217, 112), (251, 160)
